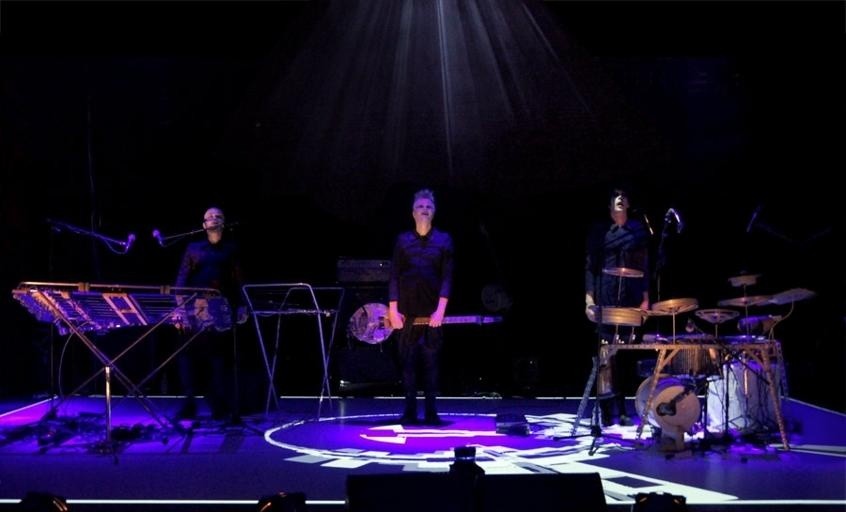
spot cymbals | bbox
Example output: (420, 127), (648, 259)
(695, 309), (738, 324)
(718, 295), (771, 306)
(603, 267), (645, 278)
(772, 289), (814, 305)
(726, 275), (758, 286)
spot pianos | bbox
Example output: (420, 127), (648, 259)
(11, 282), (236, 335)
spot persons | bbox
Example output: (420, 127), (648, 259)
(584, 187), (648, 426)
(173, 207), (249, 421)
(389, 188), (453, 425)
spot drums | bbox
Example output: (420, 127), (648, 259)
(666, 334), (724, 382)
(651, 298), (698, 315)
(635, 374), (702, 434)
(601, 304), (644, 327)
(729, 340), (783, 366)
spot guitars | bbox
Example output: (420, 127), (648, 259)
(347, 302), (513, 345)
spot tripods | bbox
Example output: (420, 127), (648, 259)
(550, 222), (643, 456)
(711, 308), (782, 443)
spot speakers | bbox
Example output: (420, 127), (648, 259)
(347, 473), (603, 512)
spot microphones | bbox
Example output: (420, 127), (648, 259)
(126, 234), (136, 251)
(643, 213), (655, 235)
(671, 209), (686, 235)
(152, 229), (163, 245)
